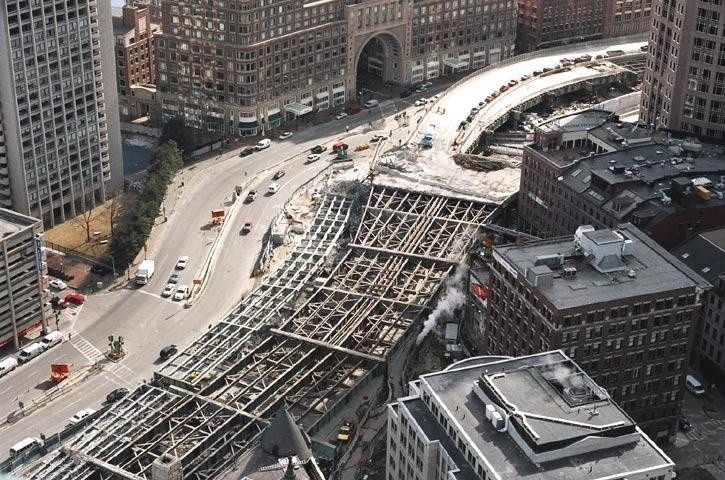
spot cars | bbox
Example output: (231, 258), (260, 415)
(159, 344), (178, 360)
(306, 131), (389, 168)
(49, 278), (86, 308)
(66, 408), (97, 427)
(108, 385), (129, 404)
(239, 168), (289, 243)
(162, 255), (188, 300)
(239, 132), (295, 162)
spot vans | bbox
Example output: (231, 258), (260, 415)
(8, 435), (43, 458)
(687, 374), (705, 396)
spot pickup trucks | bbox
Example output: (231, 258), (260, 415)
(336, 420), (355, 443)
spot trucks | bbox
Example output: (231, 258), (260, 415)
(0, 331), (63, 377)
(136, 260), (155, 284)
